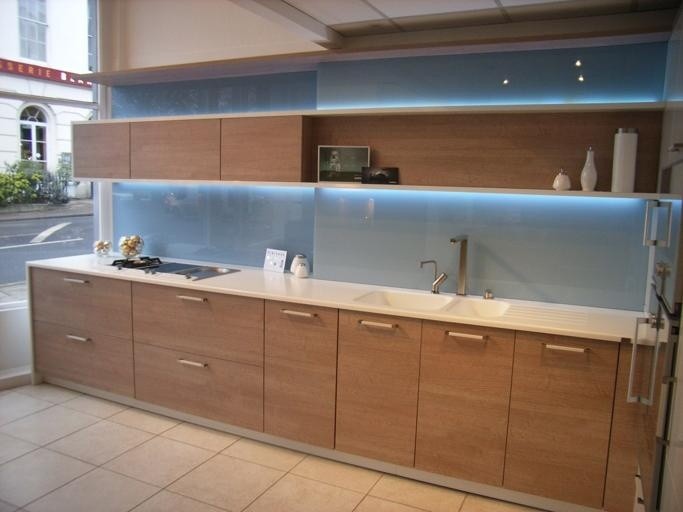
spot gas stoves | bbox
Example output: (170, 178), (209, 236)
(106, 256), (202, 275)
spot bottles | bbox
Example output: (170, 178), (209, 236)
(611, 125), (638, 192)
(580, 146), (597, 192)
(294, 263), (308, 278)
(552, 169), (571, 191)
(290, 254), (310, 274)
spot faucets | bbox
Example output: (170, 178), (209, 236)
(420, 260), (448, 294)
(450, 235), (468, 294)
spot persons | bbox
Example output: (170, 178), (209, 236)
(326, 151), (341, 180)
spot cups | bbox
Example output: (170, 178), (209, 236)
(93, 240), (112, 257)
(118, 233), (145, 259)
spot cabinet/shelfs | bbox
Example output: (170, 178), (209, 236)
(301, 106), (682, 196)
(73, 122), (131, 180)
(264, 299), (423, 469)
(132, 281), (264, 435)
(413, 319), (621, 510)
(30, 266), (135, 401)
(221, 115), (301, 182)
(131, 118), (221, 182)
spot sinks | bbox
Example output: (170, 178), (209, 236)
(436, 295), (511, 319)
(352, 290), (453, 314)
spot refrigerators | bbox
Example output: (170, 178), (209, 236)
(623, 162), (683, 512)
